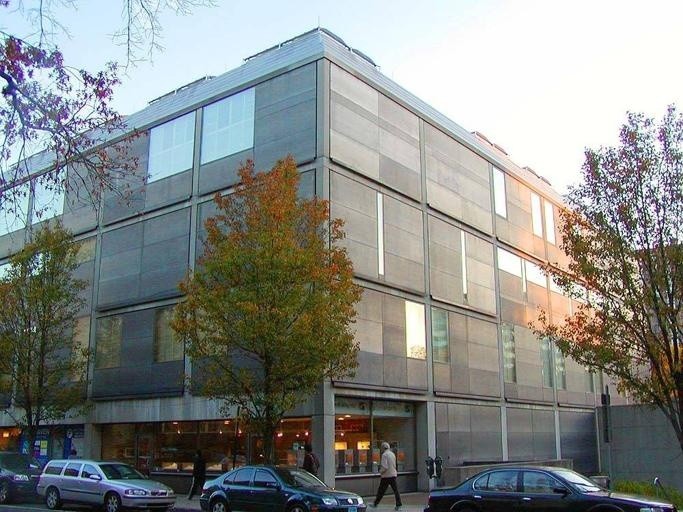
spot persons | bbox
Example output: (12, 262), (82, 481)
(68, 449), (79, 459)
(368, 441), (403, 511)
(303, 444), (320, 476)
(187, 448), (206, 500)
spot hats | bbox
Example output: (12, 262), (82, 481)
(382, 443), (390, 449)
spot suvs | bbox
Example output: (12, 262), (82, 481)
(35, 459), (176, 511)
(0, 451), (42, 504)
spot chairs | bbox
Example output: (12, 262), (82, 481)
(535, 477), (549, 493)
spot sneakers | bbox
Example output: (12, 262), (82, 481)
(395, 505), (402, 510)
(368, 503), (377, 508)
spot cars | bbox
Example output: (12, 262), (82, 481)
(33, 455), (51, 470)
(198, 465), (367, 512)
(423, 465), (675, 511)
